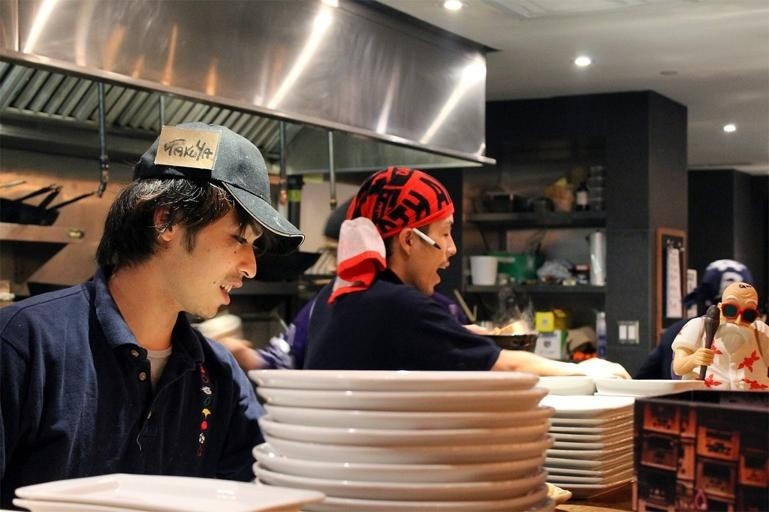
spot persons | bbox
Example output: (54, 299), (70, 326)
(672, 282), (769, 391)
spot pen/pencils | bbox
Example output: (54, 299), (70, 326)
(414, 228), (442, 252)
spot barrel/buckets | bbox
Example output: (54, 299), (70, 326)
(470, 255), (497, 286)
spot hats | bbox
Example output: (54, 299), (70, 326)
(323, 166), (454, 240)
(133, 122), (304, 256)
(700, 258), (754, 307)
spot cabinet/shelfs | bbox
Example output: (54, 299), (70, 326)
(455, 145), (608, 294)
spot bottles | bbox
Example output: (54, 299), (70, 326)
(572, 178), (592, 213)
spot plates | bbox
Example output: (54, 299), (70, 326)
(247, 369), (574, 511)
(539, 372), (712, 500)
(12, 473), (328, 510)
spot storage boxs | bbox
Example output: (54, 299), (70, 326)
(532, 309), (571, 362)
